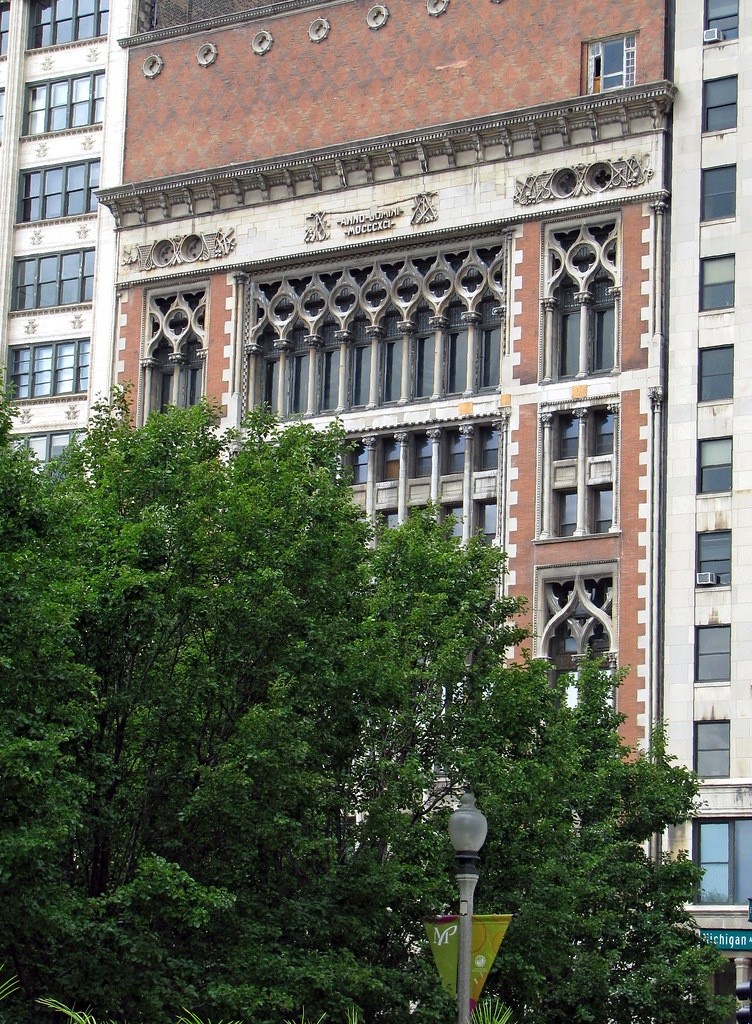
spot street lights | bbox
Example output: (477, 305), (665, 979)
(447, 791), (488, 1024)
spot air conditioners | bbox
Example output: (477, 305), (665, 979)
(696, 572), (716, 584)
(704, 28), (723, 41)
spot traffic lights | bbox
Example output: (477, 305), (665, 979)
(736, 979), (752, 1024)
